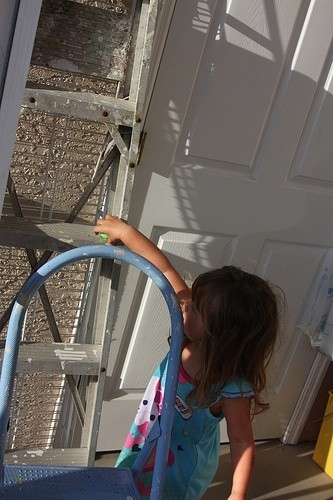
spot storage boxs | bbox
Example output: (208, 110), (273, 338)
(313, 391), (333, 482)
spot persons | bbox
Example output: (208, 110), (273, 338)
(93, 211), (287, 500)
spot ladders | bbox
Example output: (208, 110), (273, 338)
(3, 0), (160, 466)
